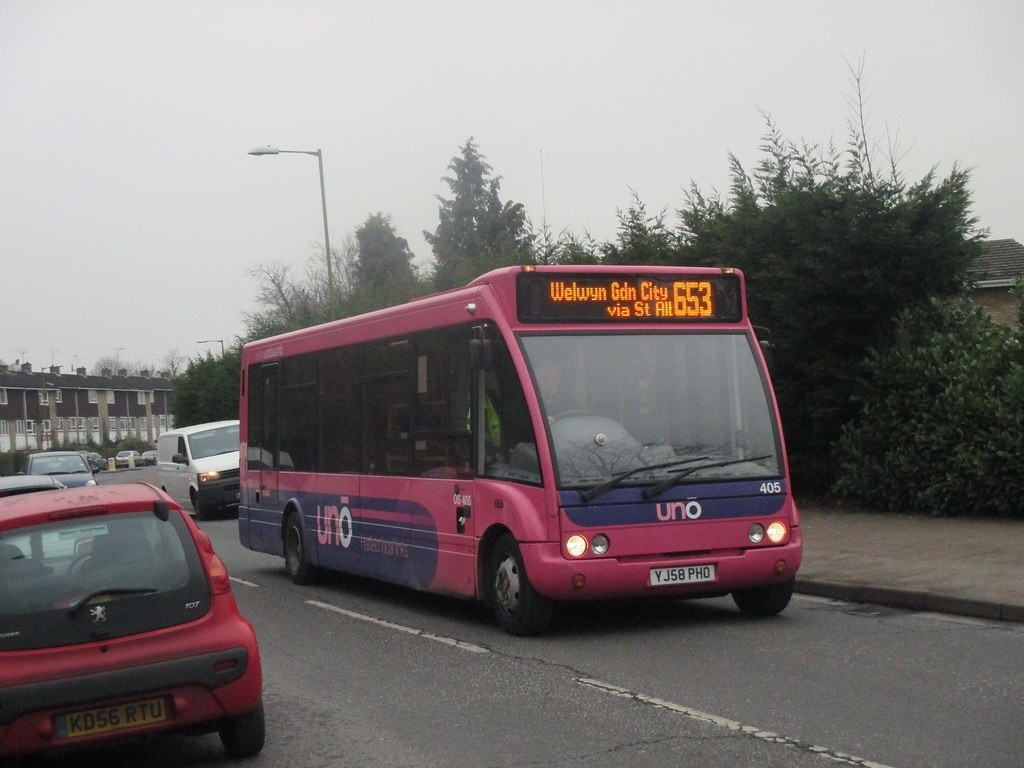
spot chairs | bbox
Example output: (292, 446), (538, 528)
(90, 532), (154, 572)
(0, 543), (34, 579)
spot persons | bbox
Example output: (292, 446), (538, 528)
(506, 369), (574, 456)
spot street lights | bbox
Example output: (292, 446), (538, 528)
(246, 144), (337, 322)
(41, 364), (65, 448)
(195, 338), (226, 356)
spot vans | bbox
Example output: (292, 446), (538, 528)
(156, 419), (241, 520)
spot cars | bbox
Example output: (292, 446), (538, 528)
(1, 481), (265, 767)
(25, 450), (97, 489)
(55, 448), (157, 477)
(0, 473), (67, 496)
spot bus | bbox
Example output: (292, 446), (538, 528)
(236, 262), (804, 635)
